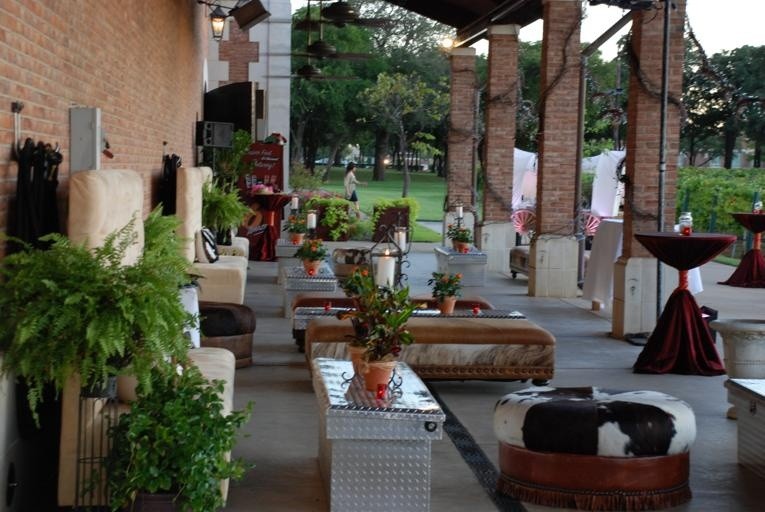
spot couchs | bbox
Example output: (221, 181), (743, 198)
(200, 167), (249, 259)
(493, 386), (697, 512)
(304, 317), (556, 387)
(57, 169), (236, 511)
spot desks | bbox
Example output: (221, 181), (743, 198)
(280, 261), (338, 319)
(635, 229), (736, 376)
(293, 306), (529, 354)
(311, 357), (446, 512)
(275, 238), (306, 284)
(434, 246), (487, 287)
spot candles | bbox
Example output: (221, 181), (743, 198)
(307, 214), (316, 229)
(376, 249), (395, 290)
(394, 232), (407, 252)
(456, 206), (463, 218)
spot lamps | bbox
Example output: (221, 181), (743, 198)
(196, 1), (227, 42)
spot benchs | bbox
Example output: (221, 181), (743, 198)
(291, 292), (495, 309)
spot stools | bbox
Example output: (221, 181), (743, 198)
(199, 300), (256, 369)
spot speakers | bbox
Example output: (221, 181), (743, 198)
(196, 121), (234, 148)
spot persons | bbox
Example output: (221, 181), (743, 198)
(343, 162), (368, 218)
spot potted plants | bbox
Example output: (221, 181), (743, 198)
(80, 361), (259, 512)
(427, 272), (464, 314)
(336, 270), (408, 373)
(360, 305), (417, 392)
(283, 214), (308, 244)
(293, 239), (331, 277)
(447, 223), (471, 253)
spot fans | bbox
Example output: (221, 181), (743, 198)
(265, 0), (357, 85)
(260, 0), (377, 62)
(268, 0), (394, 29)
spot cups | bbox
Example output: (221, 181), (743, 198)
(306, 266), (316, 277)
(375, 383), (389, 400)
(323, 302), (332, 312)
(462, 248), (470, 255)
(291, 240), (297, 245)
(470, 304), (480, 316)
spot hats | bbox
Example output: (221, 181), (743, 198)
(347, 161), (359, 168)
